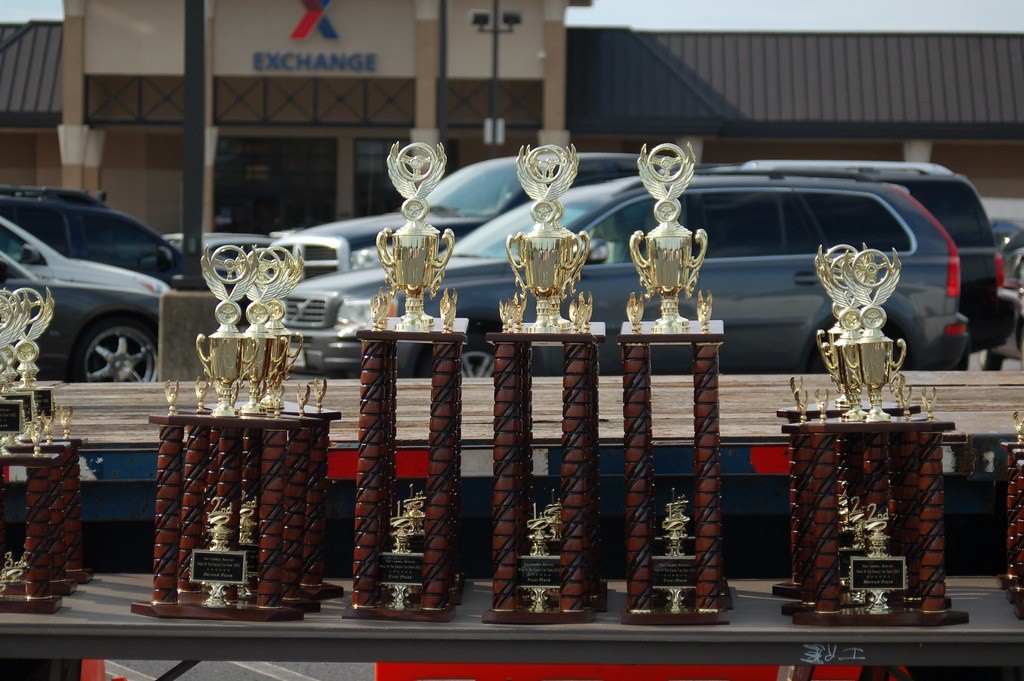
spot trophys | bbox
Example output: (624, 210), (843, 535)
(483, 138), (726, 627)
(132, 246), (347, 620)
(341, 137), (469, 617)
(998, 411), (1024, 622)
(0, 286), (90, 610)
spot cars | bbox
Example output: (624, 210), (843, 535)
(1, 220), (172, 294)
(0, 254), (162, 384)
(271, 147), (697, 295)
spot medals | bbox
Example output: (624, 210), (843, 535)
(767, 241), (972, 623)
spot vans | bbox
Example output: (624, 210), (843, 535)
(705, 161), (1015, 367)
(1, 197), (195, 287)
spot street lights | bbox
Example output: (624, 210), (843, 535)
(470, 1), (522, 160)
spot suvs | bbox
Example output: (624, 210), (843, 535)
(282, 166), (967, 375)
